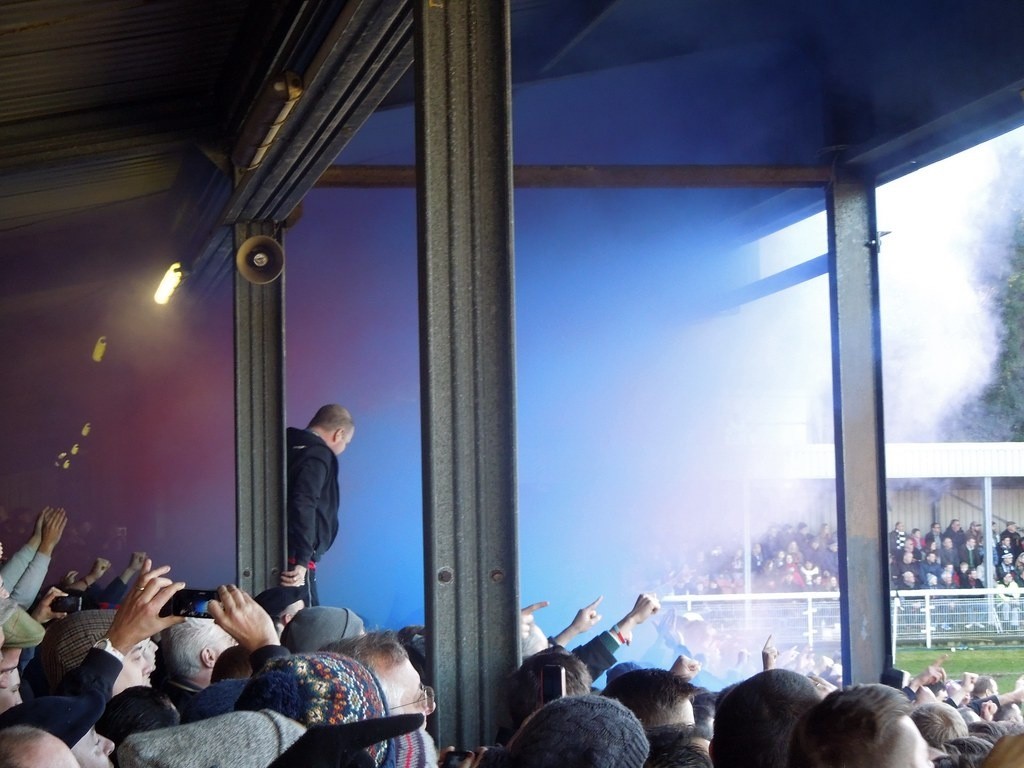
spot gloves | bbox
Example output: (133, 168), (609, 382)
(268, 712), (424, 768)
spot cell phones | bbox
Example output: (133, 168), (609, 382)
(51, 595), (83, 613)
(443, 751), (479, 768)
(541, 665), (567, 704)
(171, 589), (225, 619)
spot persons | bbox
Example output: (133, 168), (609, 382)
(0, 506), (1024, 768)
(281, 405), (355, 608)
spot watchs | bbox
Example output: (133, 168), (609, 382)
(93, 637), (125, 665)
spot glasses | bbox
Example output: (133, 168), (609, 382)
(389, 686), (434, 711)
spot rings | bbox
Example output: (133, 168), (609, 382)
(815, 682), (820, 687)
(136, 587), (144, 590)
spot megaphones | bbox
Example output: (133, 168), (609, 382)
(235, 236), (285, 284)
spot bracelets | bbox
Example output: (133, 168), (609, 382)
(79, 577), (88, 588)
(613, 625), (632, 646)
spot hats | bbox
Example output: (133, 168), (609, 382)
(2, 605), (46, 647)
(280, 606), (364, 655)
(234, 658), (397, 768)
(253, 585), (308, 620)
(0, 689), (106, 751)
(117, 708), (307, 768)
(508, 693), (651, 768)
(41, 609), (117, 695)
